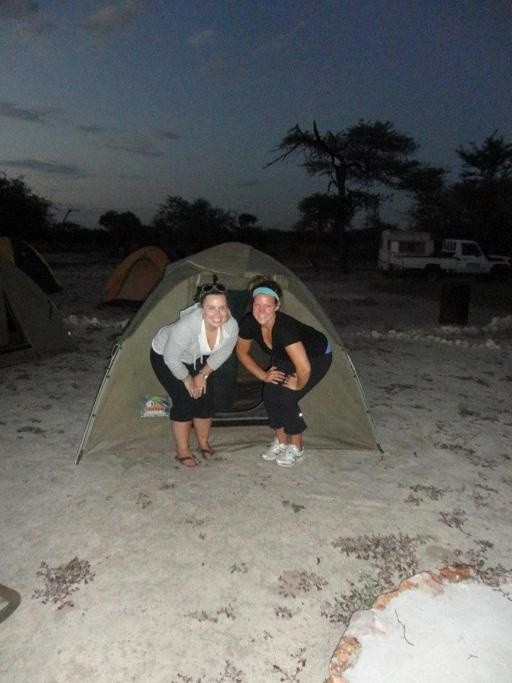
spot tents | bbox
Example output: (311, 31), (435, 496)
(0, 257), (80, 369)
(75, 242), (385, 466)
(100, 245), (172, 302)
(0, 236), (63, 295)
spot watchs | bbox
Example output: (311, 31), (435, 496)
(199, 370), (209, 379)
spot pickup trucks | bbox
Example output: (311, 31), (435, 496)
(390, 237), (510, 273)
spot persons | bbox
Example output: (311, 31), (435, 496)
(150, 284), (239, 467)
(236, 274), (332, 468)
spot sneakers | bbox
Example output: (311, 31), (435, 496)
(276, 445), (306, 466)
(260, 440), (286, 461)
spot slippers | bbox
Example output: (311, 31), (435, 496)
(174, 452), (200, 467)
(198, 441), (217, 461)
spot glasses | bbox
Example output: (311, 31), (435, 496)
(199, 283), (227, 296)
